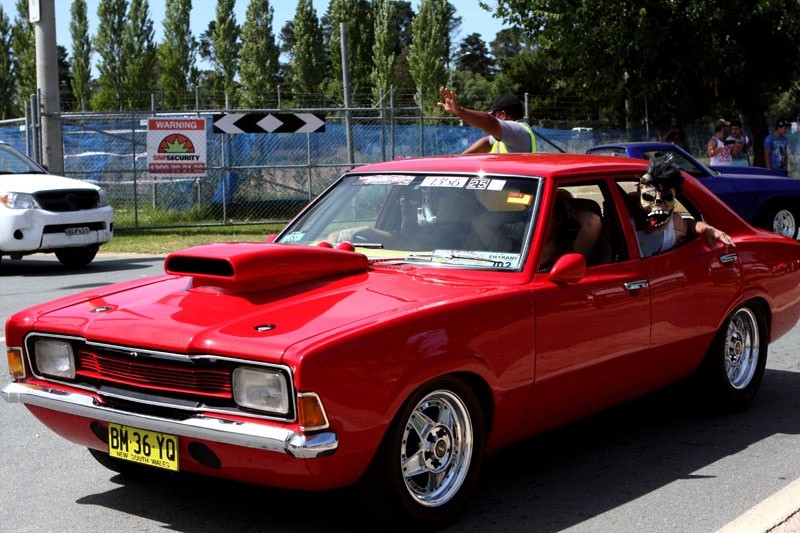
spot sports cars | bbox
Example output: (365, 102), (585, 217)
(0, 155), (800, 519)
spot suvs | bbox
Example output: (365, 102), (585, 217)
(0, 141), (115, 267)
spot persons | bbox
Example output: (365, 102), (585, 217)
(326, 189), (430, 251)
(621, 157), (744, 259)
(725, 120), (755, 167)
(706, 118), (733, 160)
(763, 118), (792, 176)
(436, 85), (537, 153)
(665, 119), (692, 155)
(472, 189), (588, 269)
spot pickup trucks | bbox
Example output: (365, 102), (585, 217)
(585, 142), (800, 238)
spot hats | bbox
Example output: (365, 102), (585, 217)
(776, 119), (791, 127)
(487, 94), (523, 121)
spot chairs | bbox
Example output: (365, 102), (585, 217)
(573, 199), (610, 266)
(423, 186), (484, 251)
